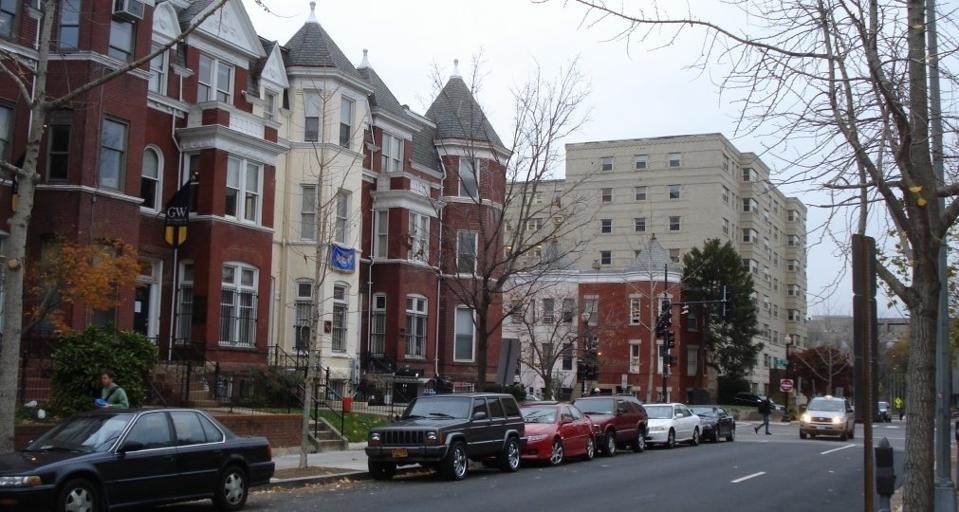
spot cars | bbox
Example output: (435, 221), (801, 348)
(518, 401), (602, 466)
(687, 404), (738, 442)
(800, 395), (855, 441)
(733, 392), (785, 410)
(878, 401), (892, 423)
(0, 408), (275, 512)
(641, 403), (704, 447)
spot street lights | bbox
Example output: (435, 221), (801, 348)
(581, 311), (591, 397)
(781, 333), (792, 422)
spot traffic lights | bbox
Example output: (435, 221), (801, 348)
(656, 313), (676, 368)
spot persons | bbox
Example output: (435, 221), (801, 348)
(100, 369), (129, 409)
(755, 397), (772, 435)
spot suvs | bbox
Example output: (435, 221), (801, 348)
(364, 392), (529, 482)
(571, 396), (650, 459)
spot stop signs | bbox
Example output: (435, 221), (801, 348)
(779, 378), (794, 392)
